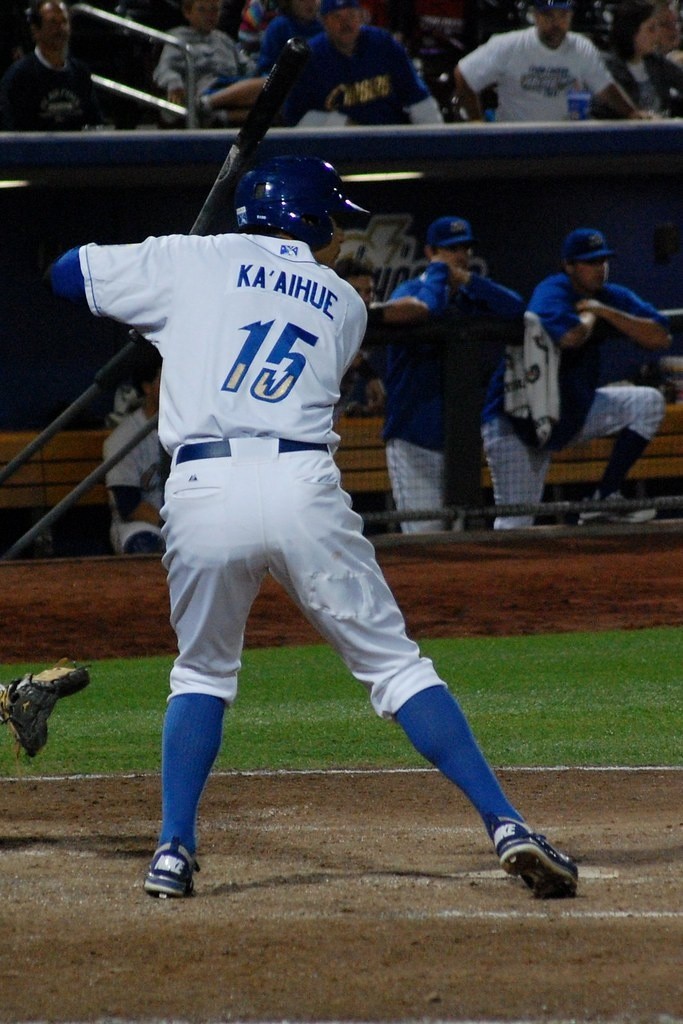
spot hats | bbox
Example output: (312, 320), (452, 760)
(535, 0), (571, 11)
(560, 227), (614, 259)
(320, 0), (358, 14)
(426, 216), (474, 246)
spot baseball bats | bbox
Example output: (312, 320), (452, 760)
(186, 38), (312, 238)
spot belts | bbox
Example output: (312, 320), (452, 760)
(176, 437), (328, 465)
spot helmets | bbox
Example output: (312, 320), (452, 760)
(234, 153), (371, 249)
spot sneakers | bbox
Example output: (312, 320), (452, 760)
(578, 489), (657, 525)
(143, 836), (200, 899)
(484, 812), (578, 898)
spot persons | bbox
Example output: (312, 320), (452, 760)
(100, 354), (171, 555)
(336, 217), (674, 535)
(49, 153), (580, 899)
(0, 0), (683, 130)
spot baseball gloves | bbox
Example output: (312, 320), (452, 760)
(0, 655), (96, 763)
